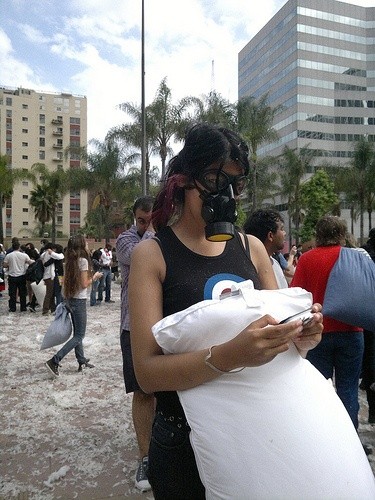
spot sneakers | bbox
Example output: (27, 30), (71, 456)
(46, 357), (62, 378)
(135, 456), (152, 490)
(79, 363), (95, 371)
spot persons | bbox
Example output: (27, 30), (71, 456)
(115, 194), (159, 490)
(45, 233), (104, 379)
(343, 228), (375, 427)
(288, 216), (373, 454)
(88, 244), (120, 305)
(244, 206), (317, 289)
(128, 126), (325, 500)
(0, 237), (68, 316)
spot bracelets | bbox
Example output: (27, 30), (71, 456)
(94, 274), (98, 278)
(204, 344), (246, 375)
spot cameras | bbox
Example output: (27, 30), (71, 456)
(279, 307), (316, 336)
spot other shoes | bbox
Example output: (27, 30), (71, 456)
(21, 308), (27, 311)
(29, 307), (36, 313)
(105, 300), (115, 303)
